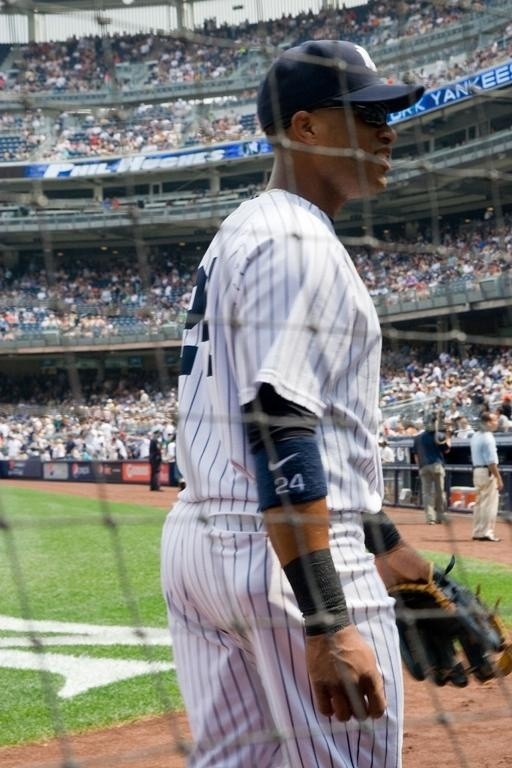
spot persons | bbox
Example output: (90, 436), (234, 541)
(0, 1), (512, 163)
(1, 347), (511, 462)
(413, 415), (451, 525)
(1, 140), (511, 341)
(158, 39), (511, 766)
(167, 432), (186, 491)
(470, 412), (503, 541)
(148, 430), (164, 492)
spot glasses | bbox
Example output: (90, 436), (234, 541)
(329, 101), (388, 128)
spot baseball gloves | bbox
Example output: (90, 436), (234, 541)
(387, 562), (512, 687)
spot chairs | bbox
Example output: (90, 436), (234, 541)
(2, 0), (511, 464)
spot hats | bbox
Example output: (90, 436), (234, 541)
(255, 39), (425, 133)
